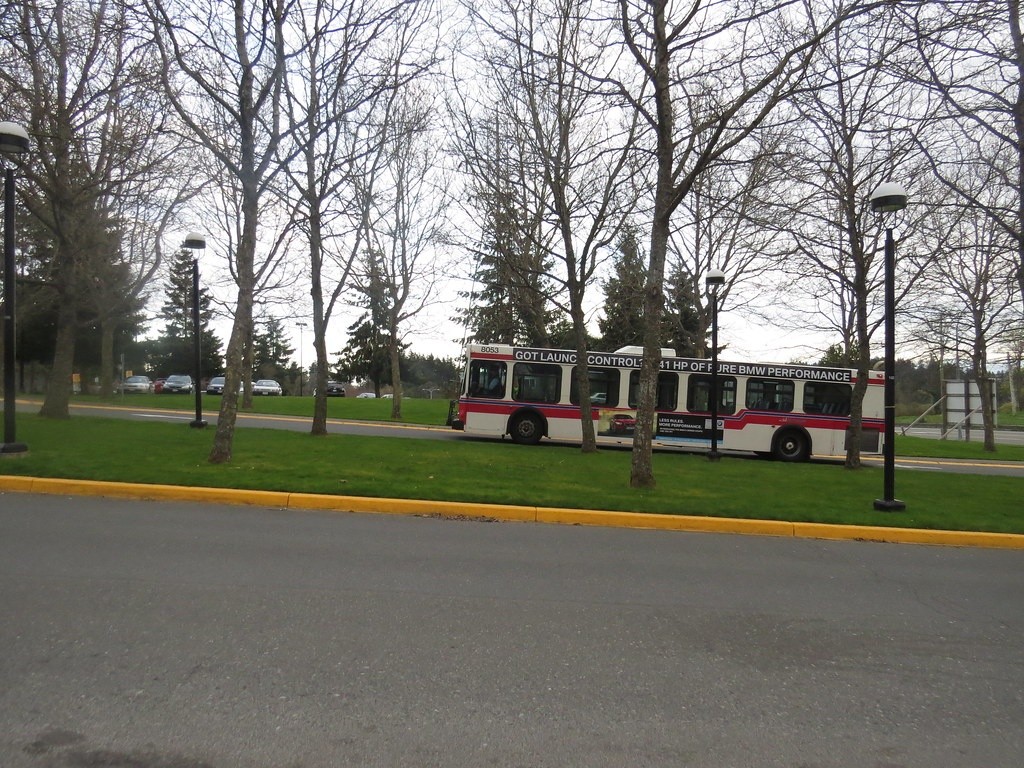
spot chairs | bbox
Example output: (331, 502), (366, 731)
(752, 398), (850, 416)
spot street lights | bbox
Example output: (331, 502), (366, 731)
(705, 270), (726, 460)
(295, 322), (307, 396)
(183, 232), (206, 428)
(873, 181), (907, 512)
(0, 122), (29, 453)
(423, 389), (440, 398)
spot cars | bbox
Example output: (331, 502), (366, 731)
(117, 375), (167, 394)
(162, 374), (195, 394)
(313, 381), (346, 398)
(239, 381), (255, 393)
(207, 377), (225, 395)
(381, 394), (393, 398)
(589, 392), (607, 405)
(252, 379), (282, 396)
(357, 393), (375, 399)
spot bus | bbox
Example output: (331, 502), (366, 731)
(445, 343), (885, 461)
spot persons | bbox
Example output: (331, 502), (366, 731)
(477, 365), (502, 395)
